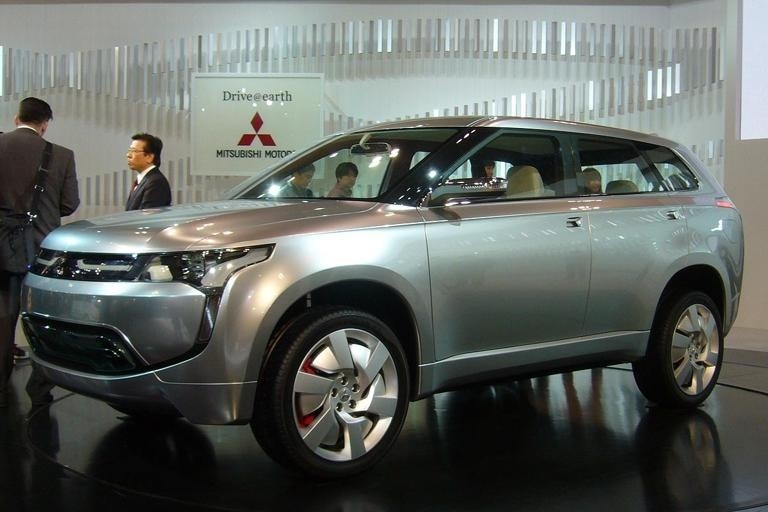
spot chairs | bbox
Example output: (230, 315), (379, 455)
(605, 179), (638, 194)
(503, 166), (544, 200)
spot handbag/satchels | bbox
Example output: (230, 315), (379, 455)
(0, 142), (53, 277)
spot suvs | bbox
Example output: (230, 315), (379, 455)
(17, 116), (745, 486)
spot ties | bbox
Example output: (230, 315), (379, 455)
(129, 178), (139, 199)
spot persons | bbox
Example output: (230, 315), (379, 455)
(124, 133), (171, 211)
(583, 167), (603, 194)
(326, 162), (358, 198)
(0, 97), (80, 405)
(281, 164), (315, 197)
(483, 160), (495, 177)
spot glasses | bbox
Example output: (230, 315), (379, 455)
(128, 149), (151, 157)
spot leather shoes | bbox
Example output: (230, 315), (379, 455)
(12, 344), (26, 358)
